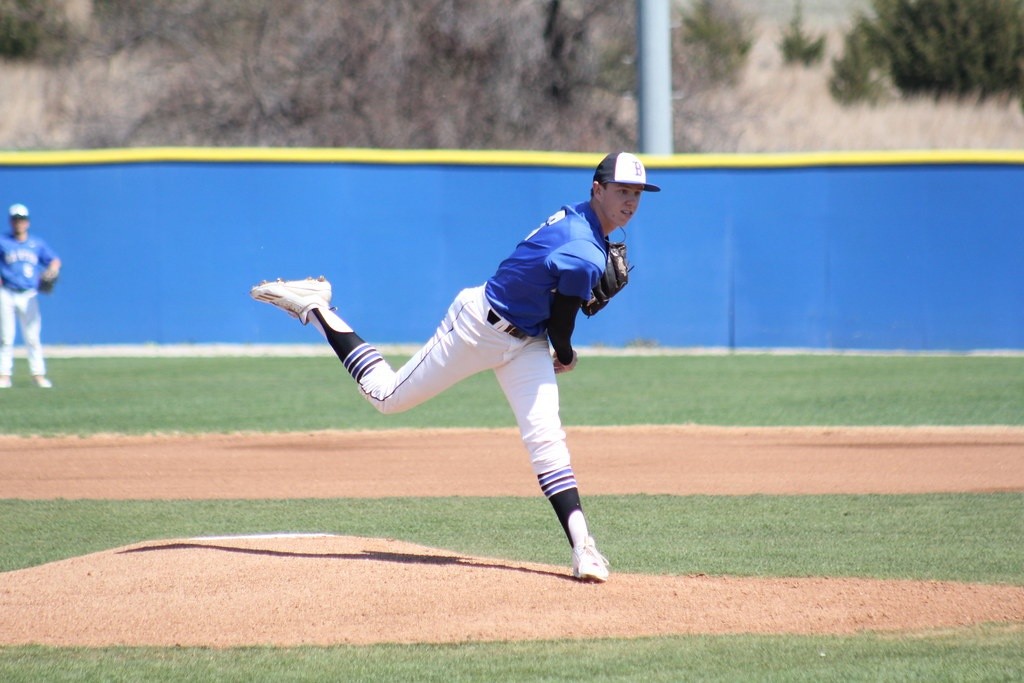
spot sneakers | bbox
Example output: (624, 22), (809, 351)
(249, 275), (331, 325)
(572, 537), (609, 581)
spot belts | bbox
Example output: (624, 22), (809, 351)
(487, 309), (527, 339)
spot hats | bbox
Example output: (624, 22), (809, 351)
(9, 203), (29, 218)
(593, 152), (661, 193)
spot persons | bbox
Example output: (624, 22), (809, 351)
(0, 203), (61, 388)
(248, 151), (661, 583)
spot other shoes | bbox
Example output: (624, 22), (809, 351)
(0, 378), (12, 388)
(32, 378), (53, 389)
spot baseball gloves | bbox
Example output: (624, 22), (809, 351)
(583, 240), (635, 318)
